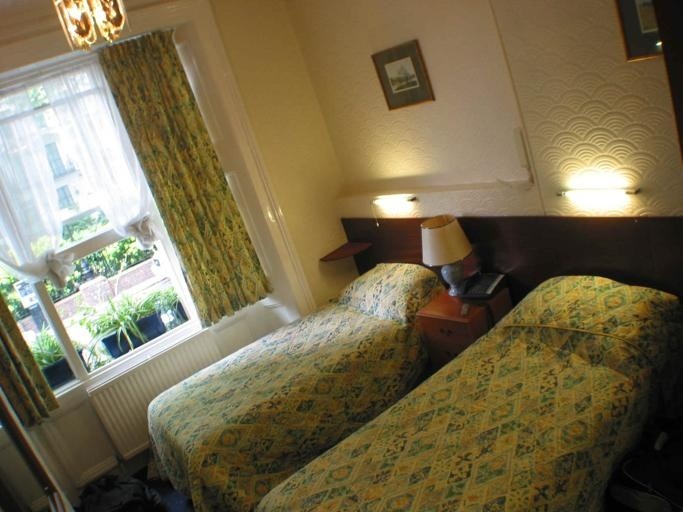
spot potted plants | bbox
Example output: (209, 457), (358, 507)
(28, 285), (190, 391)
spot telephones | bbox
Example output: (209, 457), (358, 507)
(460, 272), (505, 300)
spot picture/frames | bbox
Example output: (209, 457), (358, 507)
(613, 0), (665, 64)
(369, 38), (436, 112)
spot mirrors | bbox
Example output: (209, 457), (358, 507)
(0, 398), (69, 512)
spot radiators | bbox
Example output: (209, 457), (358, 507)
(84, 326), (227, 467)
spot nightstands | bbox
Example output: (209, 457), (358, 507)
(414, 286), (512, 375)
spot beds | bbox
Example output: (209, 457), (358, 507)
(257, 274), (682, 512)
(144, 262), (445, 512)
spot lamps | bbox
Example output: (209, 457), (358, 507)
(369, 196), (415, 205)
(555, 187), (641, 198)
(420, 213), (474, 298)
(52, 0), (132, 56)
